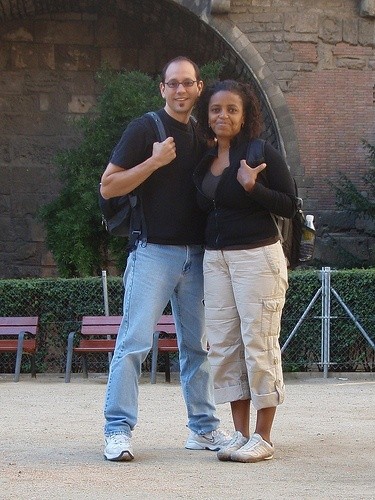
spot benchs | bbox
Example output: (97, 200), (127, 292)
(0, 317), (39, 382)
(65, 314), (210, 384)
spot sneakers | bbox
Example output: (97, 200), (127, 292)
(104, 434), (134, 462)
(185, 428), (232, 450)
(231, 433), (275, 462)
(217, 430), (249, 461)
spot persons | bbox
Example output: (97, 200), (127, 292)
(101, 57), (233, 461)
(193, 81), (299, 462)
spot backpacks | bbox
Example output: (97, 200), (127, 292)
(246, 135), (316, 268)
(97, 112), (172, 251)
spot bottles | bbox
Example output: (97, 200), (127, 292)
(298, 215), (315, 261)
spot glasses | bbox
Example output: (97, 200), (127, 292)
(164, 80), (199, 88)
